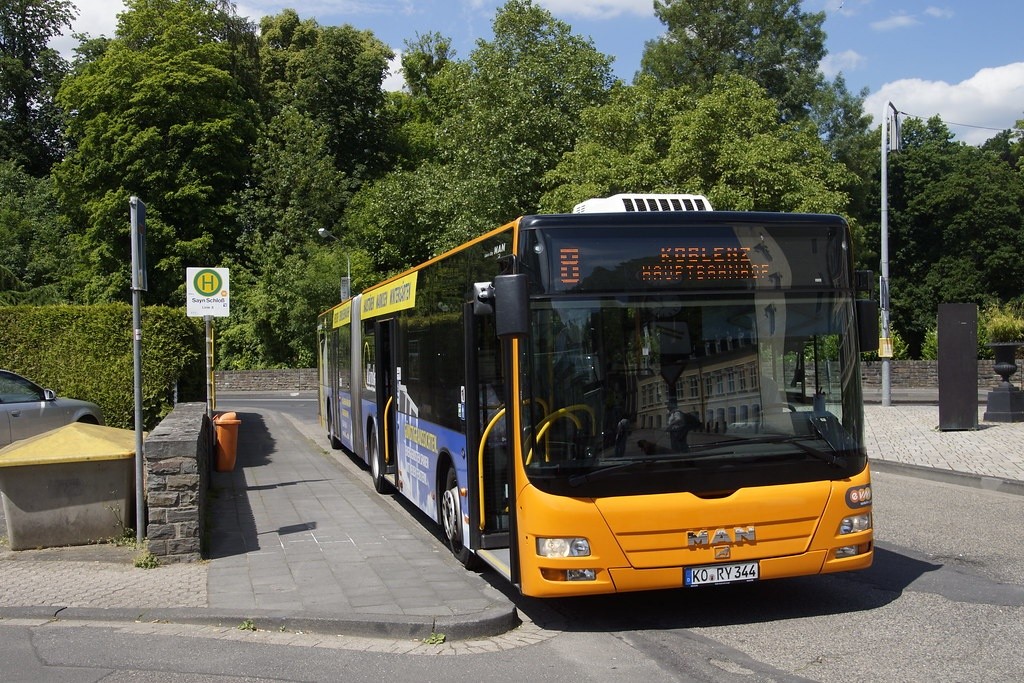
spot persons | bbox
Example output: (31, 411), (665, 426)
(614, 412), (637, 457)
(665, 397), (688, 453)
(574, 408), (590, 459)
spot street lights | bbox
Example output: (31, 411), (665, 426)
(318, 227), (351, 300)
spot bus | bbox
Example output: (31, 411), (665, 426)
(318, 191), (877, 601)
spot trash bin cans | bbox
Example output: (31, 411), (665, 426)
(213, 412), (241, 473)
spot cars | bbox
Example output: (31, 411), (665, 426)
(0, 369), (106, 450)
